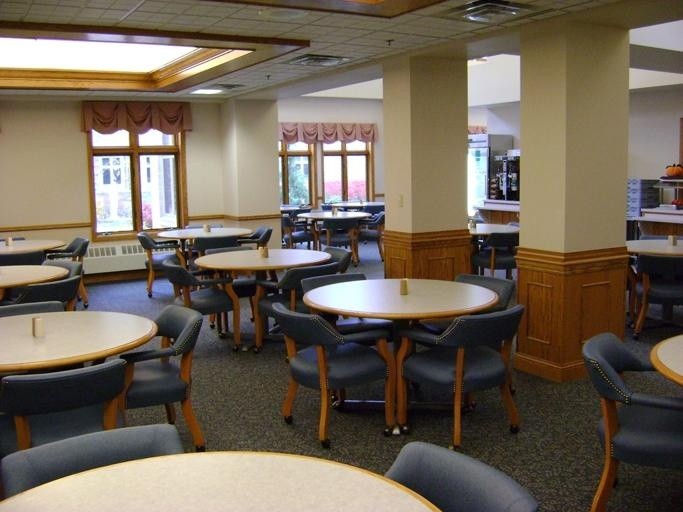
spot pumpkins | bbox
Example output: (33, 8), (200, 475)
(665, 166), (683, 176)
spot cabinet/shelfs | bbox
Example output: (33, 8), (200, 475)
(627, 179), (660, 217)
